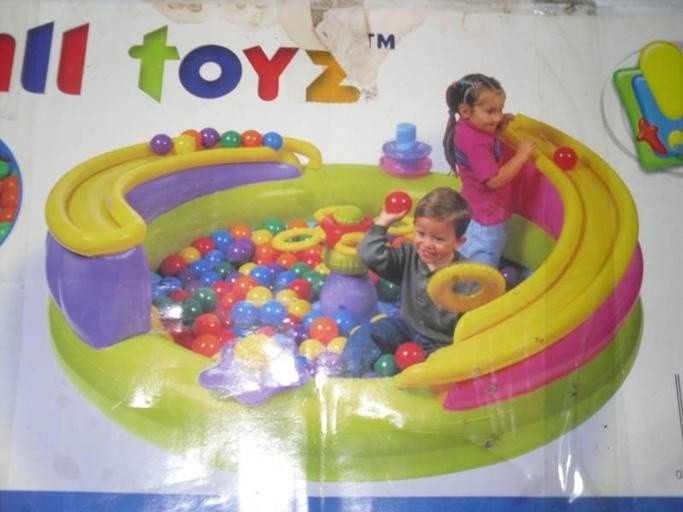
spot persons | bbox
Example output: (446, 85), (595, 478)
(441, 71), (537, 270)
(334, 184), (486, 380)
